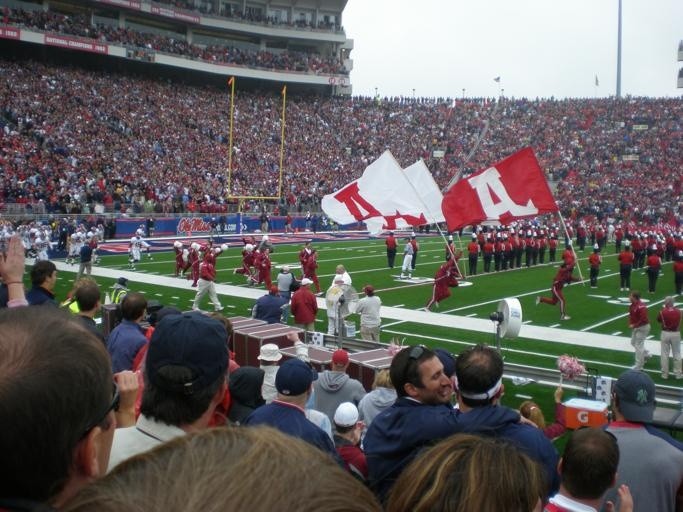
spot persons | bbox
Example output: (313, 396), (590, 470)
(0, 0), (681, 512)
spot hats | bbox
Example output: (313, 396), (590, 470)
(146, 312), (227, 391)
(363, 285), (373, 292)
(274, 359), (318, 396)
(615, 370), (655, 423)
(257, 344), (283, 362)
(270, 266), (344, 292)
(333, 349), (348, 365)
(334, 402), (359, 426)
